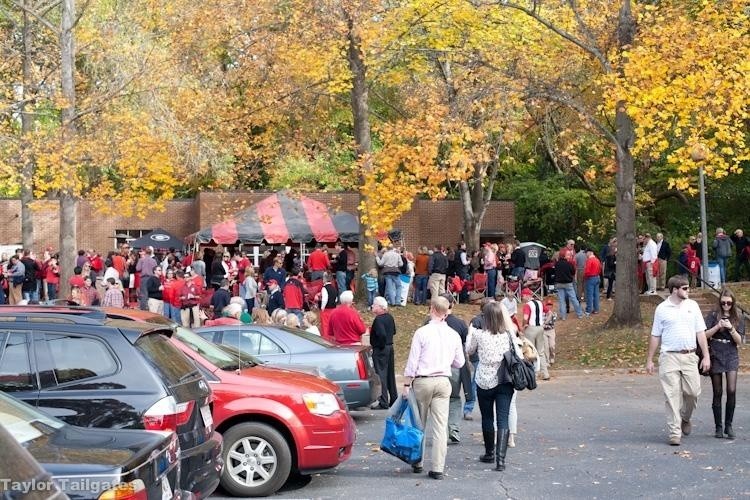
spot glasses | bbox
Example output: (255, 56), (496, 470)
(677, 286), (689, 290)
(720, 300), (732, 306)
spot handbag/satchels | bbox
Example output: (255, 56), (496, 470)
(519, 334), (539, 364)
(378, 415), (426, 468)
(697, 341), (711, 376)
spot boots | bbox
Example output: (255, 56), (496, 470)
(478, 427), (496, 464)
(724, 401), (737, 439)
(494, 428), (511, 471)
(711, 403), (724, 439)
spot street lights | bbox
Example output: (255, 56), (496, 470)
(689, 141), (714, 288)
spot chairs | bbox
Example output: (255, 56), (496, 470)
(445, 271), (544, 310)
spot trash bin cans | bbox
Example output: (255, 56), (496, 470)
(700, 261), (721, 290)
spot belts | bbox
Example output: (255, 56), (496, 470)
(711, 338), (731, 344)
(664, 347), (699, 355)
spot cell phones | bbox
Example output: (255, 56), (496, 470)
(721, 317), (727, 328)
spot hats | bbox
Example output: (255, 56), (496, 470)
(266, 279), (278, 287)
(481, 241), (506, 248)
(519, 287), (532, 295)
(544, 299), (553, 305)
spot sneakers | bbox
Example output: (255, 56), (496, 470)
(449, 430), (461, 443)
(561, 310), (599, 321)
(414, 467), (423, 473)
(428, 470), (443, 480)
(372, 403), (388, 410)
(464, 412), (473, 420)
(644, 290), (657, 295)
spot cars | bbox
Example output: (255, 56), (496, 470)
(0, 389), (200, 498)
(187, 325), (385, 419)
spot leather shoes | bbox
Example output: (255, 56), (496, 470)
(682, 419), (691, 435)
(668, 436), (682, 446)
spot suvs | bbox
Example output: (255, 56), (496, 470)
(0, 296), (357, 498)
(0, 306), (227, 499)
